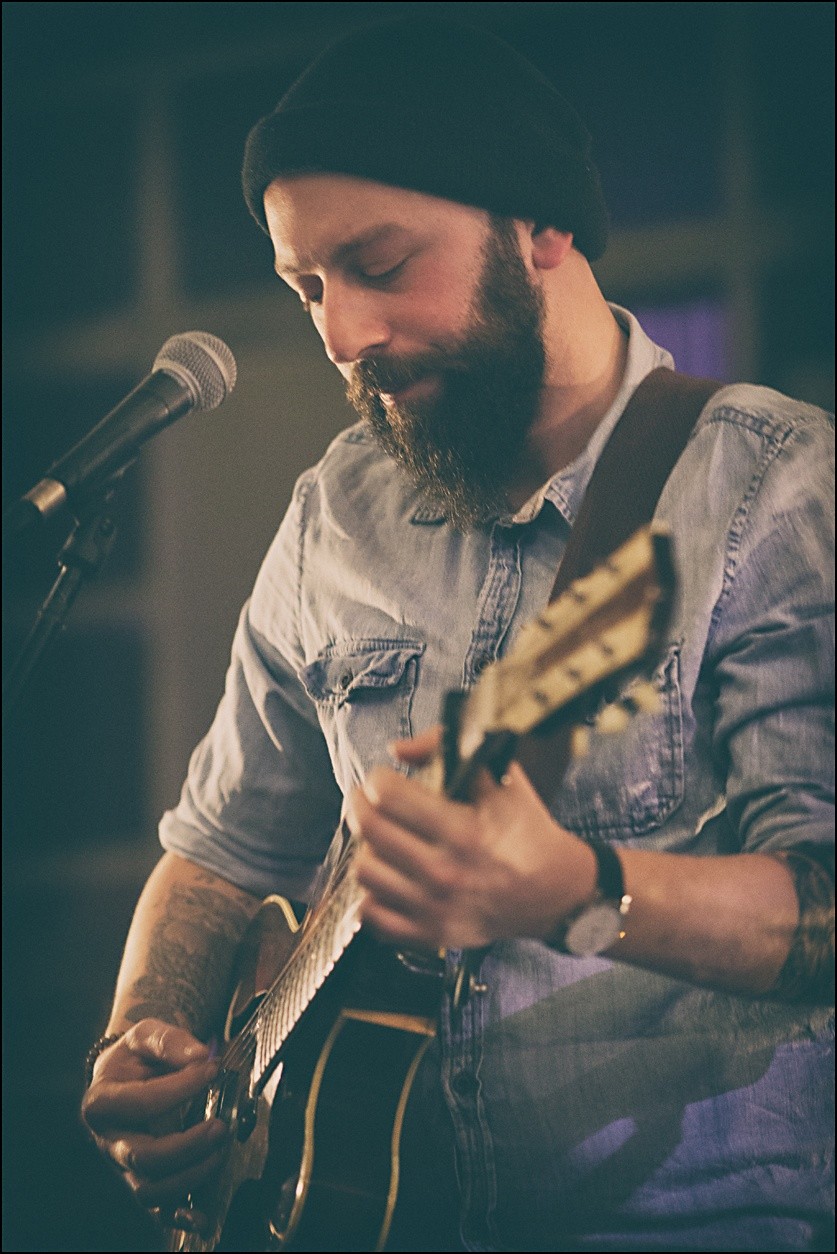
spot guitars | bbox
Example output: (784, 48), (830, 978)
(163, 519), (689, 1254)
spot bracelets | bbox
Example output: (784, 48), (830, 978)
(84, 1032), (125, 1090)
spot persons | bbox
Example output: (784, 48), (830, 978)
(82, 6), (837, 1253)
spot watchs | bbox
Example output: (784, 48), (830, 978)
(543, 833), (629, 958)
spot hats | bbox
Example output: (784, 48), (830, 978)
(243, 18), (608, 261)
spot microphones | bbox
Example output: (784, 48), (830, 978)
(11, 331), (237, 531)
